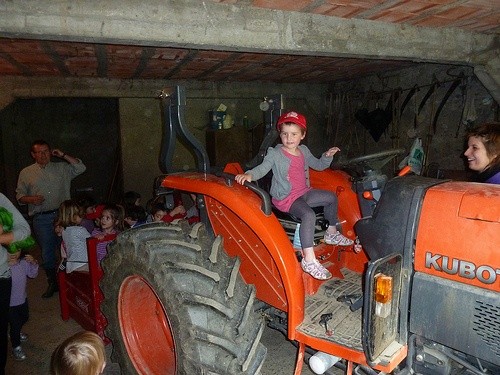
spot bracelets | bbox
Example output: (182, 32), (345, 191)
(62, 153), (65, 159)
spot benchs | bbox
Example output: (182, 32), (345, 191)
(59, 217), (201, 345)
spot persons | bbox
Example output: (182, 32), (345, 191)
(464, 122), (500, 184)
(16, 139), (86, 298)
(0, 192), (39, 375)
(234, 112), (354, 280)
(50, 331), (107, 375)
(55, 191), (201, 274)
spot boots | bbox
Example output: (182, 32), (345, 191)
(41, 267), (59, 298)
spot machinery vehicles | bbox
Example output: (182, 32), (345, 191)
(99, 81), (500, 373)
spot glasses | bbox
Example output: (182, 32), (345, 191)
(32, 149), (52, 155)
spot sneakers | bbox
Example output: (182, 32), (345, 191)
(9, 346), (27, 360)
(18, 332), (32, 344)
(300, 258), (333, 281)
(322, 230), (353, 247)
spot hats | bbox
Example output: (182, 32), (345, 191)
(277, 110), (307, 131)
(85, 204), (105, 220)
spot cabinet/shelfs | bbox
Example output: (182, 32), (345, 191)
(206, 126), (247, 168)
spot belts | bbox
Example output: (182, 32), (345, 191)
(34, 209), (59, 215)
